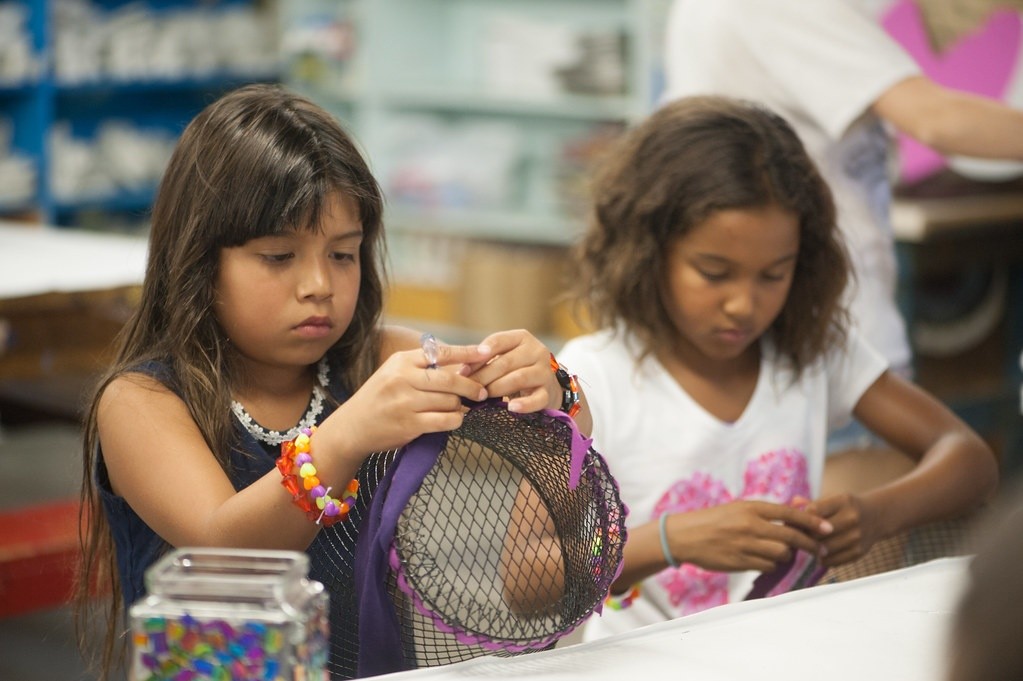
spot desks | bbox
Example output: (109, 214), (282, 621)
(352, 550), (973, 681)
(0, 219), (151, 375)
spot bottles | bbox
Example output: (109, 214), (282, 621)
(129, 546), (328, 681)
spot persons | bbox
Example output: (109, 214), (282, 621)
(663, 1), (1023, 587)
(499, 92), (998, 650)
(94, 80), (594, 680)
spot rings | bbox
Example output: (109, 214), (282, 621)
(420, 330), (440, 367)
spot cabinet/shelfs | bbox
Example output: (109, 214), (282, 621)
(0, 0), (669, 253)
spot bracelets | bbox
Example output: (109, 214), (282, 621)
(531, 352), (580, 441)
(279, 423), (360, 527)
(660, 510), (681, 569)
